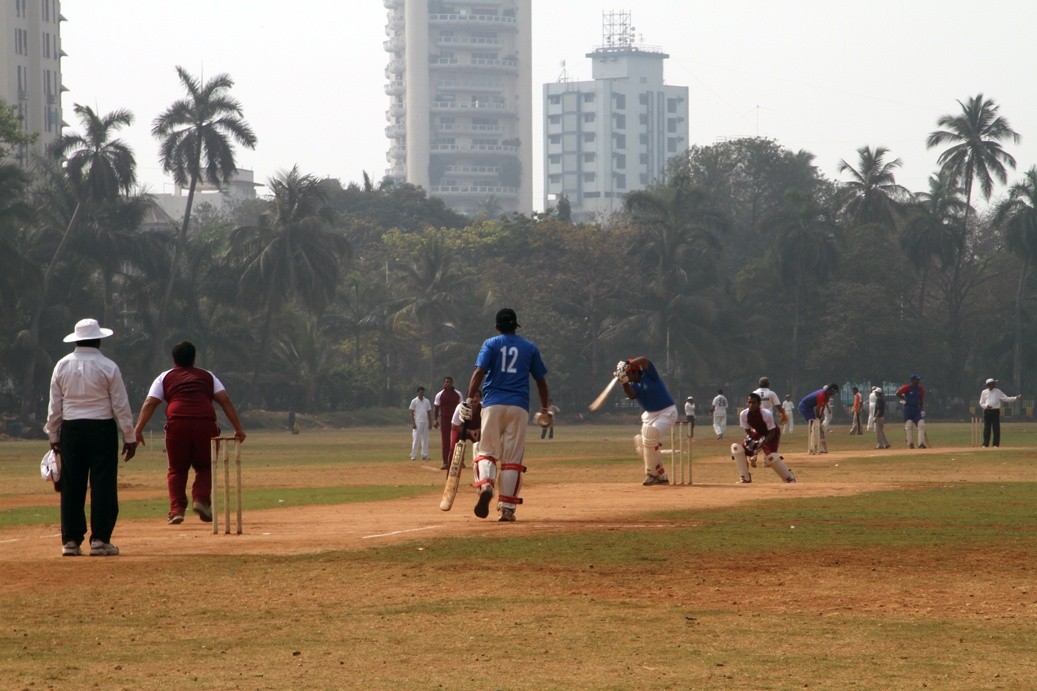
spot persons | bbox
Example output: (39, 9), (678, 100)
(42, 318), (137, 557)
(849, 387), (864, 435)
(730, 393), (796, 484)
(541, 398), (561, 439)
(684, 396), (696, 438)
(134, 341), (247, 525)
(866, 385), (877, 432)
(750, 377), (789, 468)
(979, 378), (1021, 448)
(409, 386), (432, 460)
(433, 376), (466, 470)
(446, 389), (483, 493)
(872, 387), (892, 449)
(782, 395), (795, 433)
(710, 389), (728, 440)
(287, 406), (296, 431)
(821, 398), (833, 434)
(459, 307), (553, 523)
(797, 384), (840, 453)
(613, 356), (679, 486)
(895, 372), (927, 448)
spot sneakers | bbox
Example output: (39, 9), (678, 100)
(90, 541), (120, 557)
(642, 474), (658, 485)
(62, 542), (80, 555)
(654, 478), (669, 486)
(167, 515), (183, 524)
(497, 509), (516, 522)
(192, 501), (212, 522)
(473, 486), (492, 519)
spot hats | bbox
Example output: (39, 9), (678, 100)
(495, 308), (522, 329)
(687, 396), (694, 400)
(40, 450), (61, 491)
(985, 378), (1000, 385)
(911, 374), (923, 379)
(63, 318), (114, 343)
(759, 376), (769, 386)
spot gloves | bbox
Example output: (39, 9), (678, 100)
(900, 400), (906, 404)
(921, 410), (926, 417)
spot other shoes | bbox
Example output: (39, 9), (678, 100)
(993, 445), (998, 447)
(982, 445), (987, 448)
(681, 425), (941, 459)
(407, 448), (468, 470)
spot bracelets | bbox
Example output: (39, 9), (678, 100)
(541, 407), (549, 410)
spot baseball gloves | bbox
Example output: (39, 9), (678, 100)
(743, 433), (766, 452)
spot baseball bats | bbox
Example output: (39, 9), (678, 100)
(588, 376), (619, 411)
